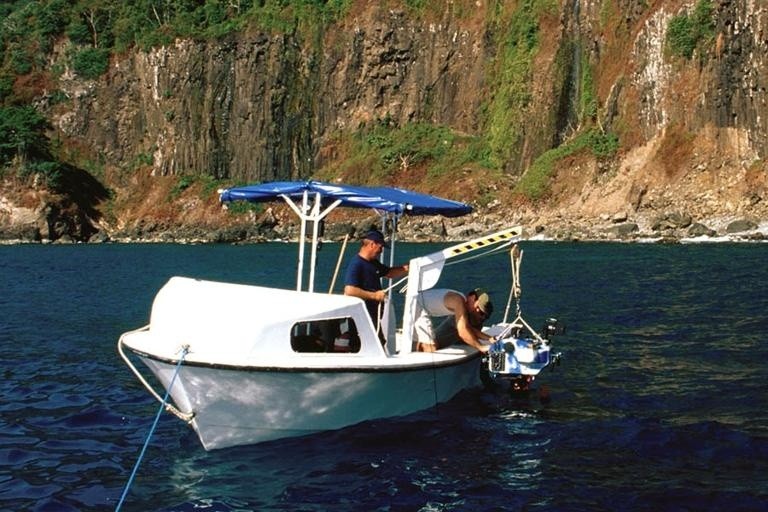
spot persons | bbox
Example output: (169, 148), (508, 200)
(414, 287), (498, 355)
(343, 230), (409, 353)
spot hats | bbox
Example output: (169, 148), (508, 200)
(364, 230), (390, 249)
(473, 287), (491, 316)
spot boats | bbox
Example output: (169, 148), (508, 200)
(122, 183), (562, 451)
